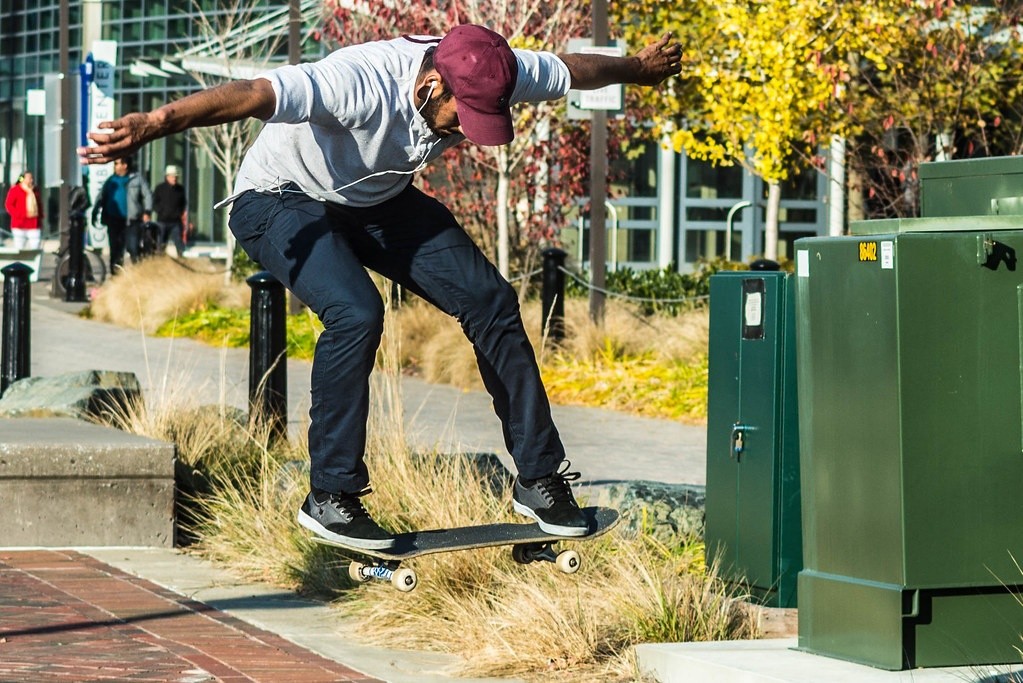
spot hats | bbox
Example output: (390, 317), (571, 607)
(162, 165), (180, 177)
(432, 24), (519, 146)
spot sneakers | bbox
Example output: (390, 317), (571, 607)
(297, 491), (396, 550)
(511, 459), (590, 536)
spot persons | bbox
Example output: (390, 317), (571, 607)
(52, 174), (93, 302)
(91, 155), (153, 275)
(152, 164), (188, 257)
(77, 23), (684, 550)
(4, 171), (44, 282)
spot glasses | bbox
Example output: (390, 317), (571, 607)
(115, 161), (125, 165)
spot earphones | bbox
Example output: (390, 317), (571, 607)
(431, 80), (438, 89)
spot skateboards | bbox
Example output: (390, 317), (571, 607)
(309, 506), (621, 592)
